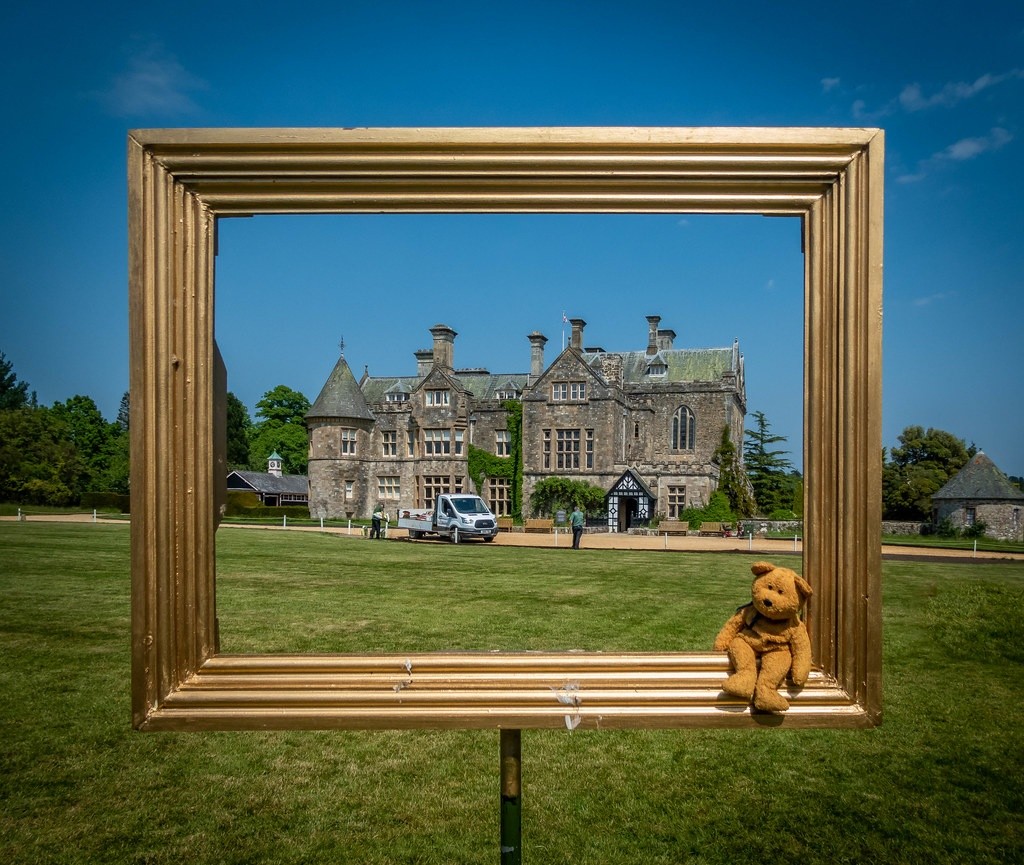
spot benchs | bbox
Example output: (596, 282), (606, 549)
(496, 518), (513, 533)
(524, 519), (554, 534)
(657, 521), (688, 536)
(699, 522), (732, 537)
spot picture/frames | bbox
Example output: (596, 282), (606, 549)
(127, 126), (885, 731)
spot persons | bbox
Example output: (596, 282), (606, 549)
(370, 503), (384, 539)
(569, 506), (584, 550)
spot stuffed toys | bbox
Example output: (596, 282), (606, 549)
(714, 562), (814, 712)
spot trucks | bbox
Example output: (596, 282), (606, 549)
(398, 492), (498, 544)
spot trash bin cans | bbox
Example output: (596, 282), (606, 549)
(744, 522), (753, 539)
